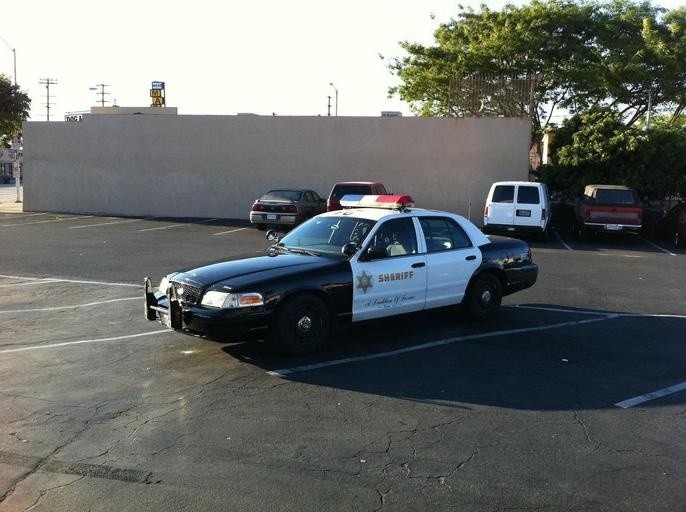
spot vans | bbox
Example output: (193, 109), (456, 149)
(324, 181), (389, 212)
(482, 180), (552, 242)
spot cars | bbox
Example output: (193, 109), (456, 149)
(142, 192), (539, 356)
(249, 188), (325, 231)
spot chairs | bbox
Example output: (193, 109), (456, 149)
(386, 231), (415, 257)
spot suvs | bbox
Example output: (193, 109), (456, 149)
(575, 184), (645, 241)
